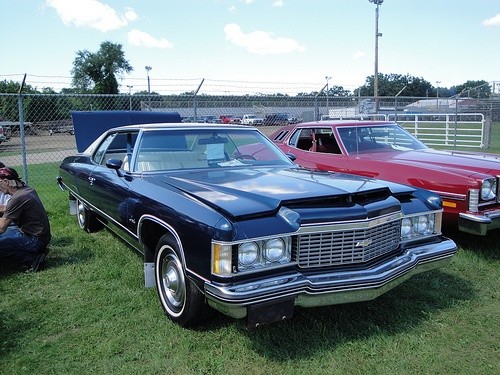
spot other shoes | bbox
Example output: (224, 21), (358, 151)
(24, 248), (50, 272)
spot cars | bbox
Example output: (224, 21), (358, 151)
(181, 112), (289, 126)
(231, 119), (500, 238)
(55, 111), (459, 333)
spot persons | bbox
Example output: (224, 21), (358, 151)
(0, 167), (51, 273)
(49, 130), (53, 136)
(0, 162), (14, 205)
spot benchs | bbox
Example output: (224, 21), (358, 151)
(122, 152), (208, 171)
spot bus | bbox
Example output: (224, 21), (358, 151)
(0, 122), (34, 136)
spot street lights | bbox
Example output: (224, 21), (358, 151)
(145, 65), (153, 112)
(369, 0), (384, 96)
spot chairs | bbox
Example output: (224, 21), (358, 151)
(313, 133), (331, 152)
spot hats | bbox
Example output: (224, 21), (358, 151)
(0, 167), (21, 181)
